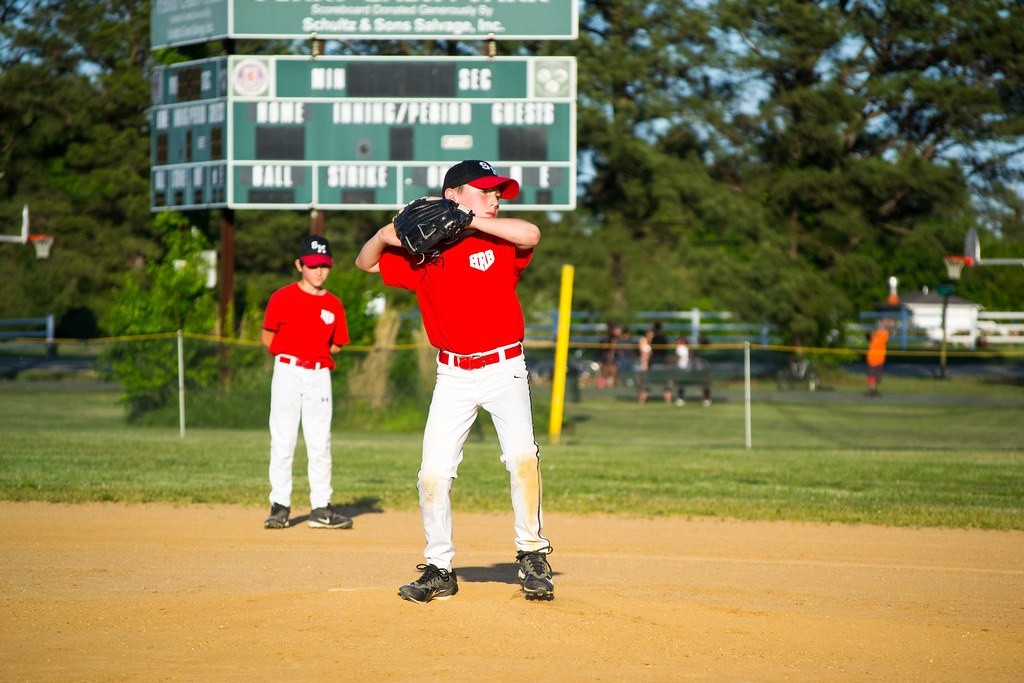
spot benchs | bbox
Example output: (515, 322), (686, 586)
(0, 316), (58, 367)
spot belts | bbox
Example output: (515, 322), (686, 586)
(438, 343), (521, 369)
(279, 357), (326, 369)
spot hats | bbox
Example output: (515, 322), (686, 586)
(299, 235), (333, 268)
(442, 159), (520, 200)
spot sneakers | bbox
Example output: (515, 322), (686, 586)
(513, 546), (555, 598)
(265, 502), (290, 528)
(398, 564), (459, 603)
(308, 503), (353, 529)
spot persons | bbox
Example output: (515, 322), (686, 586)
(355, 159), (554, 605)
(260, 235), (353, 530)
(597, 318), (713, 407)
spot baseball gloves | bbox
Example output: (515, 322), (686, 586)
(392, 193), (476, 261)
